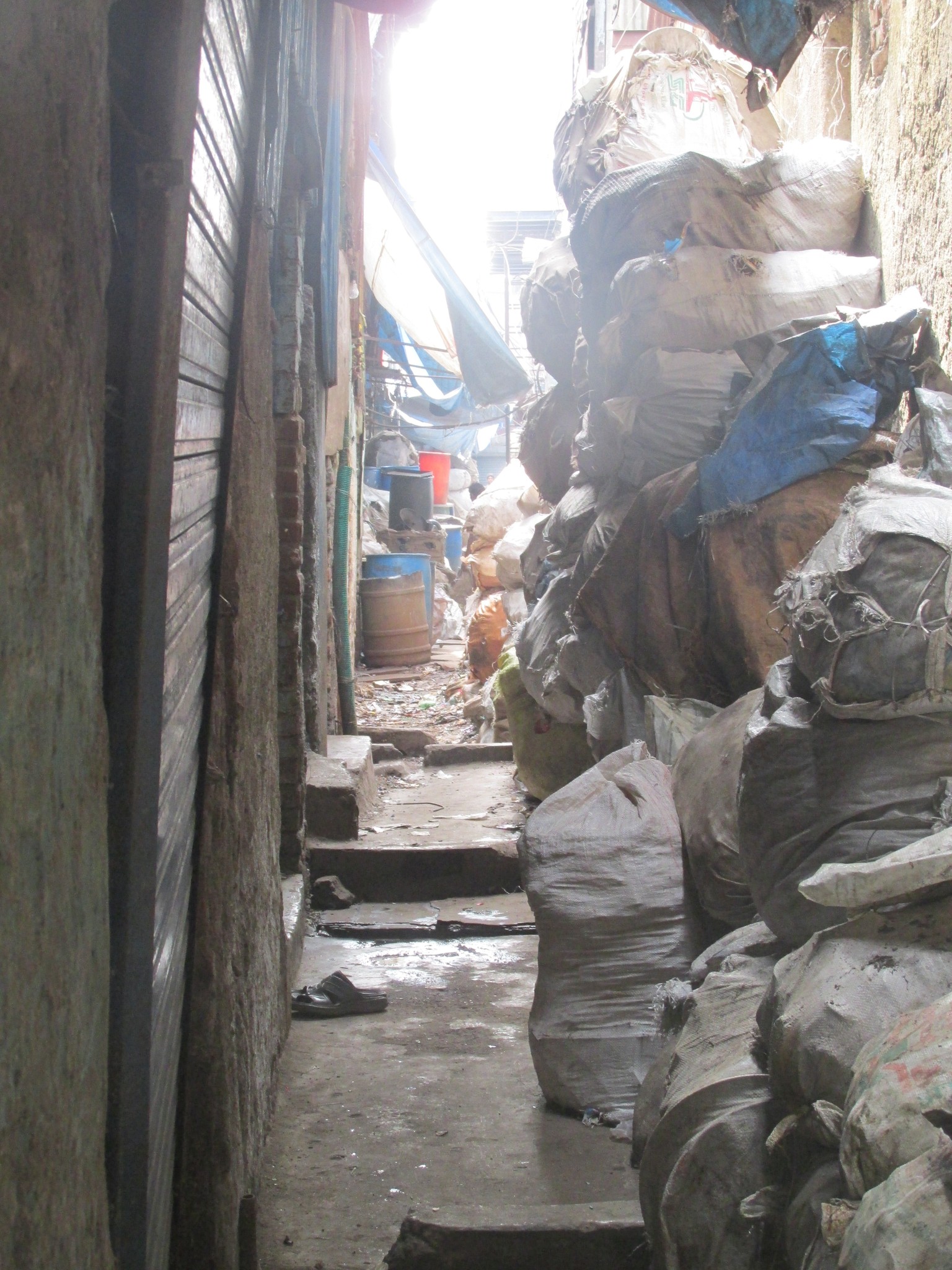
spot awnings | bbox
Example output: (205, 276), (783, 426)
(366, 140), (537, 417)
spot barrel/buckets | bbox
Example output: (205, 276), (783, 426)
(432, 502), (454, 517)
(418, 452), (451, 505)
(361, 559), (435, 621)
(364, 553), (431, 642)
(388, 474), (434, 532)
(443, 527), (462, 573)
(364, 465), (419, 493)
(359, 570), (431, 669)
